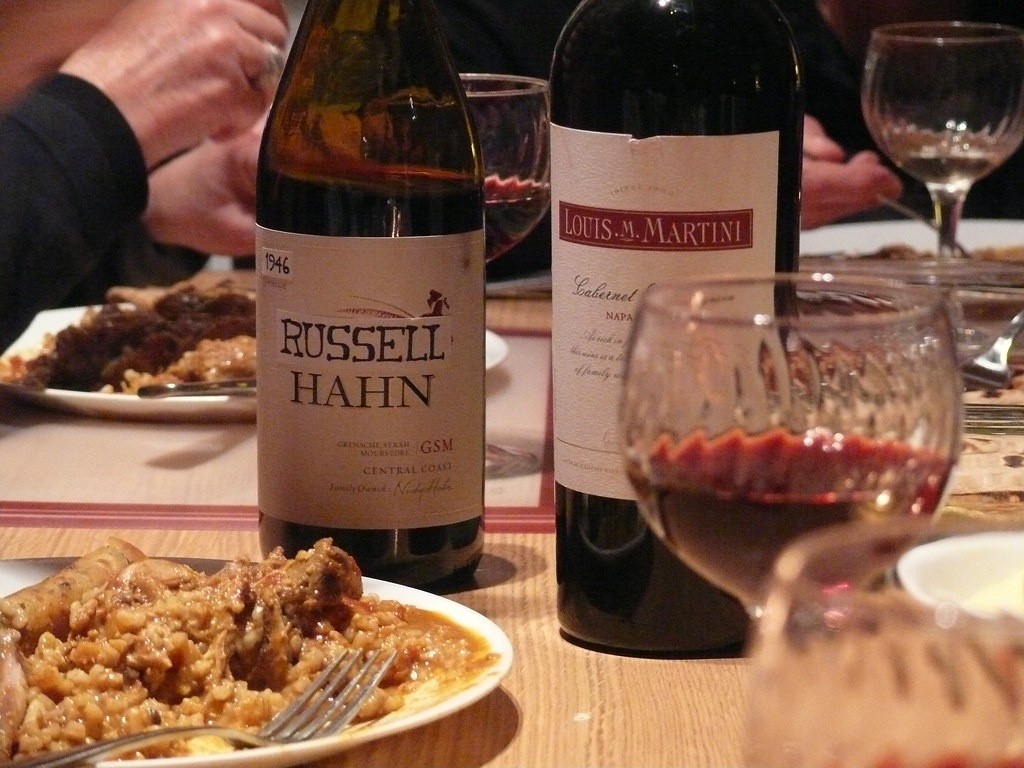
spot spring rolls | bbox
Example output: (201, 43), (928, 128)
(0, 535), (149, 653)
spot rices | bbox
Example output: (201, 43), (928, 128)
(13, 575), (428, 768)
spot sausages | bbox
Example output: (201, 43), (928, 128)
(257, 542), (363, 637)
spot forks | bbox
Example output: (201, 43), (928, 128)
(1, 649), (396, 767)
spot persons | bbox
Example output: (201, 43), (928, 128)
(396, 0), (995, 282)
(0, 0), (291, 368)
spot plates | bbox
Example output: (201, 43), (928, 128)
(1, 558), (514, 768)
(896, 531), (1024, 641)
(1, 304), (508, 424)
(798, 218), (1024, 257)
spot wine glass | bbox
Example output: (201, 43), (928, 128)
(861, 19), (1024, 257)
(617, 273), (961, 623)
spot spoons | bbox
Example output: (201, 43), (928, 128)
(957, 309), (1023, 386)
(958, 309), (1023, 393)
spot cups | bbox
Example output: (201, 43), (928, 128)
(457, 72), (548, 265)
(737, 517), (1024, 768)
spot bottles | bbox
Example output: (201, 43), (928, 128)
(254, 0), (488, 594)
(548, 0), (803, 653)
